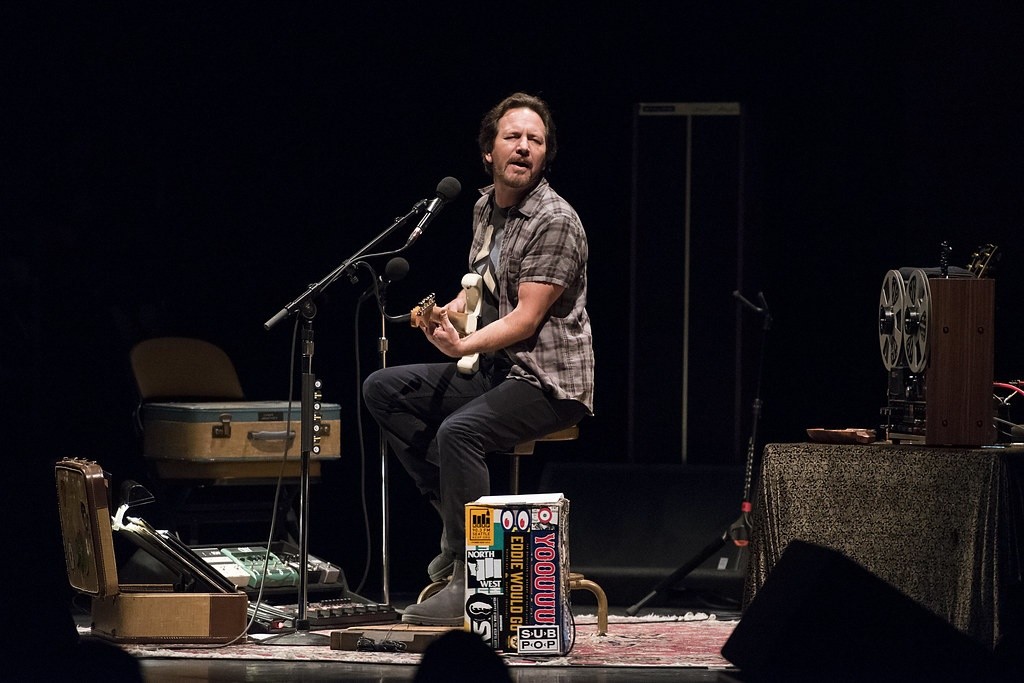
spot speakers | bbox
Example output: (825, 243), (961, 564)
(538, 462), (754, 608)
(720, 538), (1014, 683)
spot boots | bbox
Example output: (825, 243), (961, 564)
(427, 519), (452, 581)
(401, 560), (468, 625)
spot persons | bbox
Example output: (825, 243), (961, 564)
(360, 94), (595, 628)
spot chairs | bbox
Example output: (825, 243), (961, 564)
(131, 337), (320, 546)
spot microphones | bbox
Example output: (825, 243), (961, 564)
(405, 177), (461, 247)
(359, 257), (409, 303)
(728, 502), (753, 548)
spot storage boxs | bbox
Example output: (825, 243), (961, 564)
(54, 456), (248, 644)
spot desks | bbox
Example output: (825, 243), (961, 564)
(743, 444), (1024, 654)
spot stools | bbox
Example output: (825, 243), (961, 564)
(417, 426), (608, 634)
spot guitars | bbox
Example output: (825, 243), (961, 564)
(410, 272), (488, 377)
